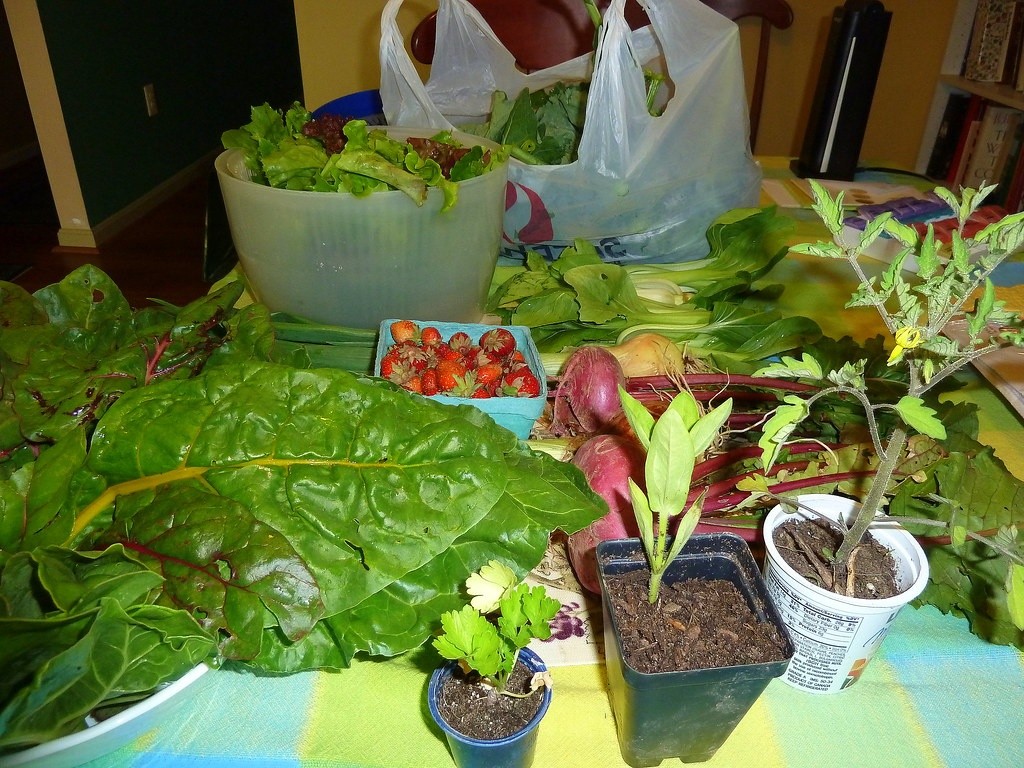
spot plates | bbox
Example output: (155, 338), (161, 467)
(0, 636), (214, 767)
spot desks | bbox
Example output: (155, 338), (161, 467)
(73, 154), (1024, 768)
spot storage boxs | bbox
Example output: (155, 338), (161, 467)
(375, 319), (547, 442)
(215, 125), (509, 329)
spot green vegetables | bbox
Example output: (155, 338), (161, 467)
(473, 203), (909, 382)
(460, 0), (668, 167)
(0, 263), (304, 754)
(301, 368), (612, 669)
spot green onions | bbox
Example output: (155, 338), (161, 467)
(266, 311), (589, 376)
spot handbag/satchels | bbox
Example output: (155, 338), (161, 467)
(379, 0), (761, 267)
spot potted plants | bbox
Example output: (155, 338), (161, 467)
(427, 560), (562, 768)
(759, 178), (1024, 692)
(594, 384), (795, 768)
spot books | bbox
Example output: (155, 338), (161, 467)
(940, 315), (1024, 418)
(926, 92), (1024, 214)
(965, 0), (1024, 92)
(833, 196), (992, 275)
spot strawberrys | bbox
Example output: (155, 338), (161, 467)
(379, 319), (540, 399)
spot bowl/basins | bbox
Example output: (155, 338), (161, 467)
(214, 125), (510, 325)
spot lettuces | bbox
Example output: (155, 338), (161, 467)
(220, 101), (506, 211)
